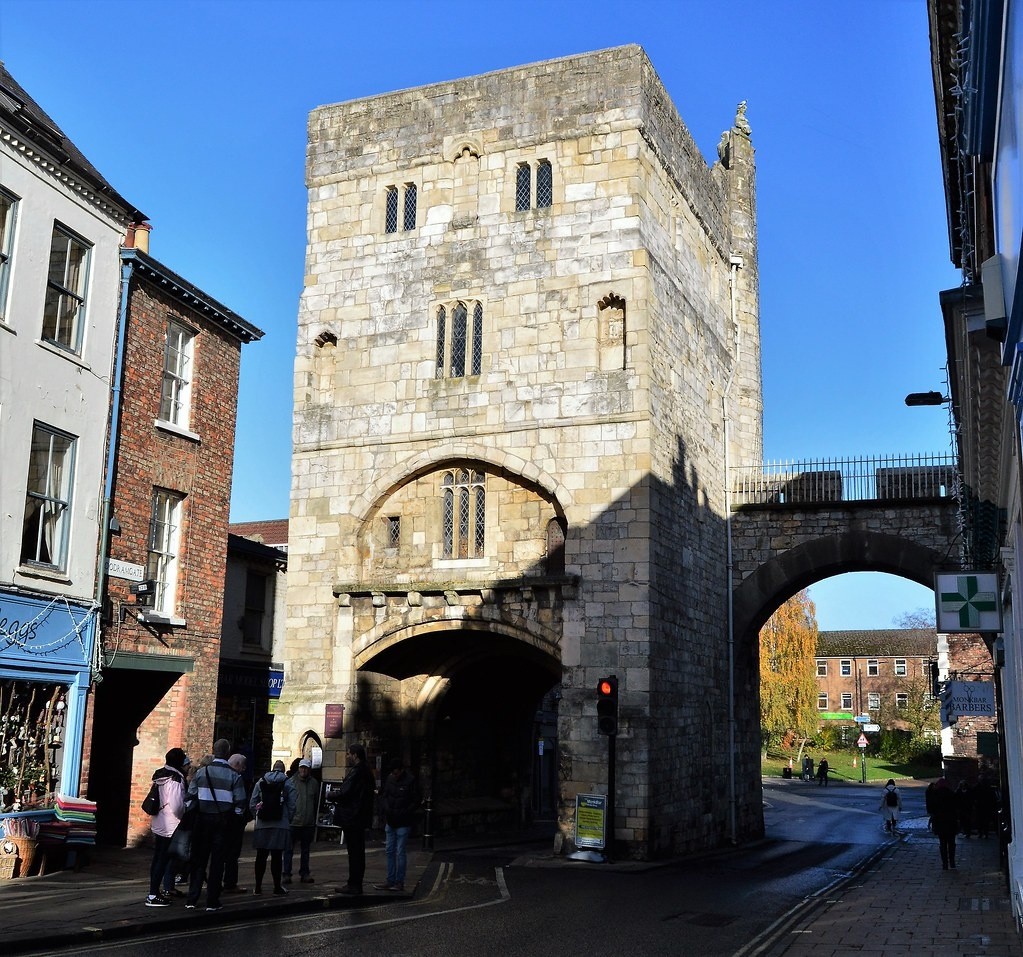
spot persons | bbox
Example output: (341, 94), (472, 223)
(802, 754), (814, 782)
(373, 754), (424, 891)
(284, 758), (318, 884)
(974, 775), (993, 839)
(927, 778), (960, 870)
(817, 758), (828, 787)
(326, 744), (377, 895)
(248, 760), (289, 897)
(954, 780), (976, 840)
(144, 740), (247, 910)
(879, 779), (902, 833)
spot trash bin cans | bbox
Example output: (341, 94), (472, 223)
(783, 766), (793, 779)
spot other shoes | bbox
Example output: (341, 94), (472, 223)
(224, 884), (247, 894)
(301, 875), (315, 883)
(272, 887), (290, 896)
(252, 888), (262, 896)
(161, 888), (183, 897)
(184, 902), (198, 909)
(282, 874), (292, 883)
(948, 855), (957, 868)
(373, 880), (404, 891)
(335, 885), (364, 895)
(942, 857), (949, 869)
(206, 904), (223, 911)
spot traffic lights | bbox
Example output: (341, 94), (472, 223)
(596, 674), (619, 737)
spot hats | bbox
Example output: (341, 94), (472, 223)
(273, 760), (285, 772)
(298, 758), (312, 768)
(183, 757), (192, 767)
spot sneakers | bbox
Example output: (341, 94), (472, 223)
(145, 893), (174, 907)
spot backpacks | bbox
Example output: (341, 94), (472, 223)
(257, 777), (290, 822)
(884, 787), (898, 806)
(142, 783), (165, 816)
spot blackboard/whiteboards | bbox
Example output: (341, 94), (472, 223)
(316, 778), (345, 829)
